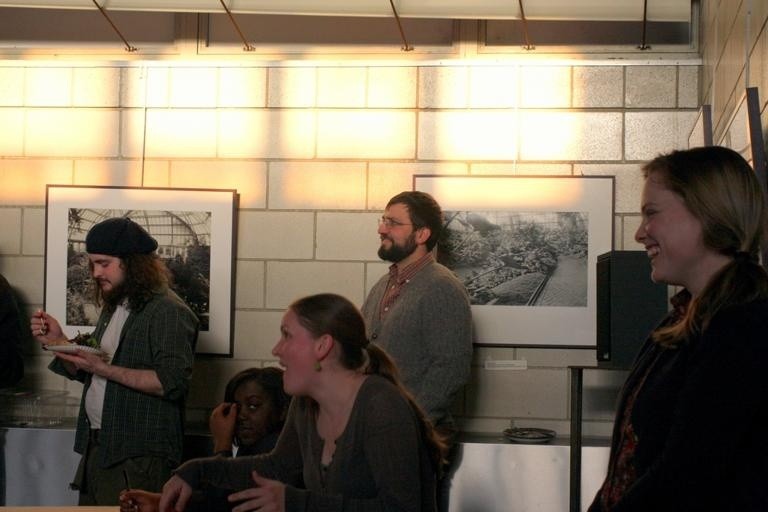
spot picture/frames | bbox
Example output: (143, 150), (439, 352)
(412, 173), (615, 350)
(43, 183), (238, 358)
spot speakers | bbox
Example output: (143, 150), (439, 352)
(596, 250), (669, 368)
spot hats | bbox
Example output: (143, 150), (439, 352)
(86, 219), (158, 257)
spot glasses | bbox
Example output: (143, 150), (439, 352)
(377, 217), (414, 227)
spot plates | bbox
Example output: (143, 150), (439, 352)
(502, 427), (556, 443)
(45, 344), (106, 356)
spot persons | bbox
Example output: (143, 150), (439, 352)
(119, 366), (291, 512)
(360, 189), (474, 466)
(586, 147), (767, 511)
(156, 293), (441, 512)
(29, 217), (201, 504)
